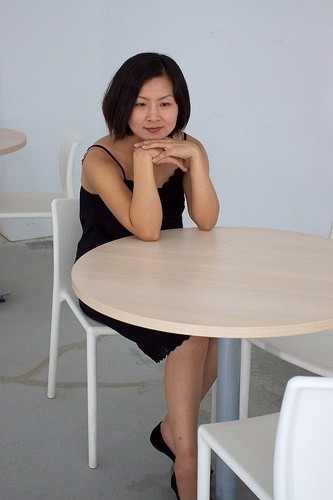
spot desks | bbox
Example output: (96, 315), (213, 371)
(69, 227), (333, 500)
(0, 127), (27, 297)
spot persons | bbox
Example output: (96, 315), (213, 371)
(72, 53), (219, 500)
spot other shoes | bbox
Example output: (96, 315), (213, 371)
(150, 421), (214, 500)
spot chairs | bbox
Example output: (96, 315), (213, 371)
(47, 196), (217, 469)
(0, 131), (78, 243)
(239, 220), (333, 420)
(197, 376), (332, 500)
